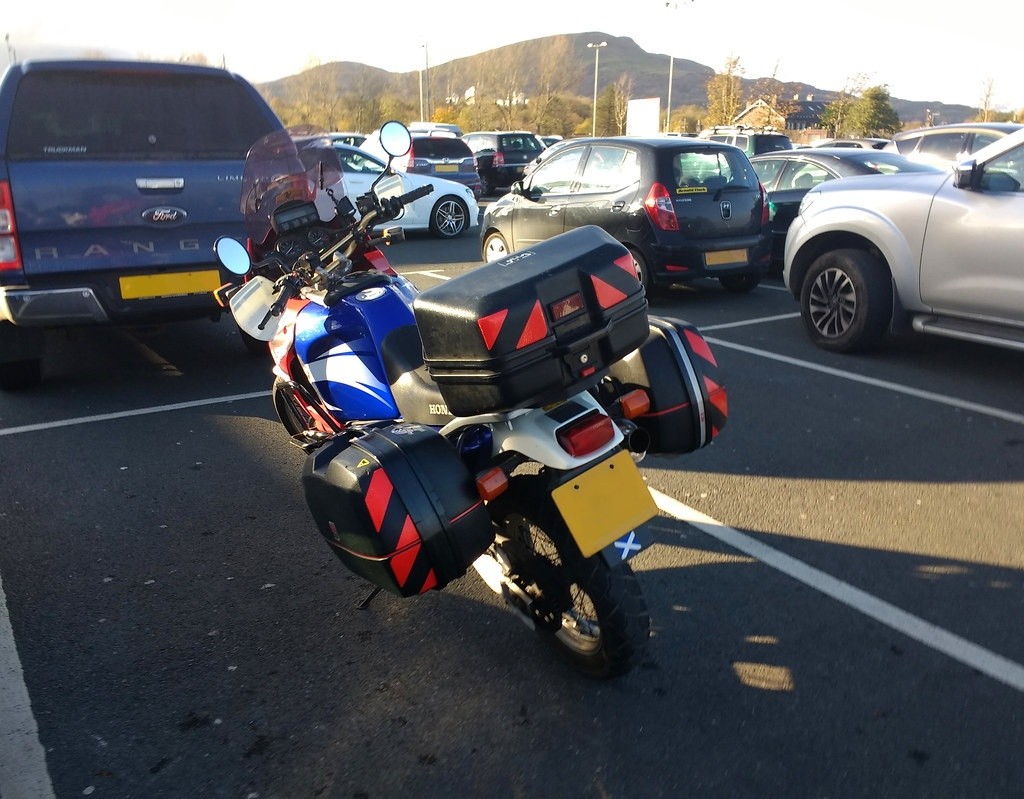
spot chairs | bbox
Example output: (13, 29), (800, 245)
(794, 173), (813, 188)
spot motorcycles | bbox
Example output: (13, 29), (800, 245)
(209, 118), (727, 684)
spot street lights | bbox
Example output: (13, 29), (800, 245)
(586, 42), (608, 139)
(665, 0), (696, 134)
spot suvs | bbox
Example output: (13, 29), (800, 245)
(352, 128), (482, 203)
(0, 56), (320, 391)
(696, 124), (793, 158)
(883, 121), (1024, 171)
(781, 126), (1024, 355)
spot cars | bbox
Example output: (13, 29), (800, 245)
(295, 142), (481, 239)
(408, 121), (463, 138)
(292, 129), (367, 149)
(520, 136), (583, 178)
(745, 148), (940, 264)
(479, 136), (774, 302)
(459, 130), (549, 197)
(658, 131), (699, 137)
(816, 136), (890, 149)
(511, 134), (562, 148)
